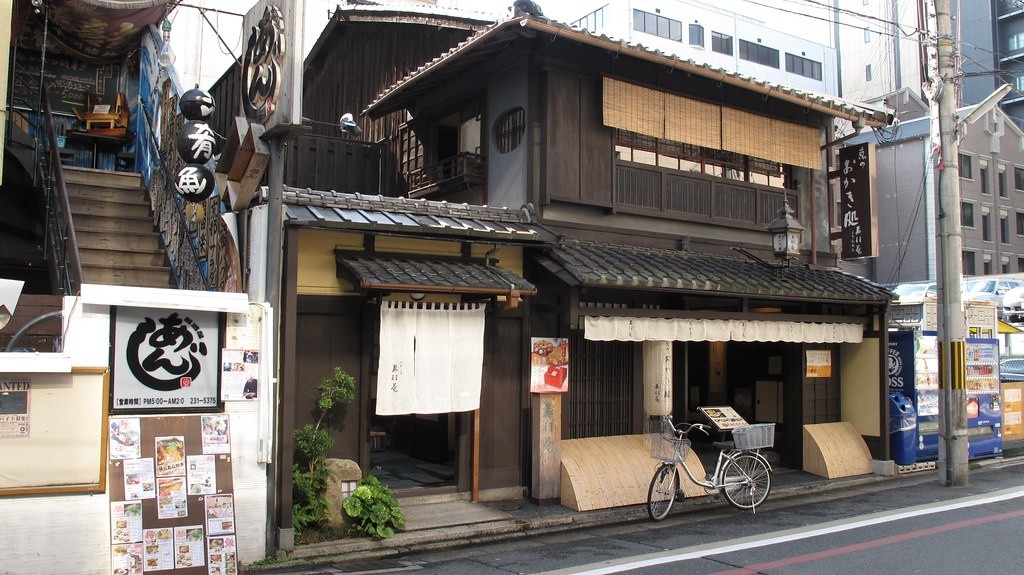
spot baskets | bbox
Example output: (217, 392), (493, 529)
(649, 433), (691, 464)
(731, 421), (775, 449)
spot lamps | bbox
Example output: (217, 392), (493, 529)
(767, 190), (804, 268)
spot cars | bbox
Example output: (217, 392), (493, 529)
(1003, 286), (1024, 322)
(1000, 354), (1023, 382)
(891, 282), (942, 302)
(962, 279), (1023, 297)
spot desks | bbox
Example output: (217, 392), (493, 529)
(66, 132), (127, 170)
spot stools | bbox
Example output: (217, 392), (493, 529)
(117, 151), (136, 171)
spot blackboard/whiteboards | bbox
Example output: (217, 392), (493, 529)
(0, 366), (110, 498)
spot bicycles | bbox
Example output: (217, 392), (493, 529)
(646, 414), (774, 522)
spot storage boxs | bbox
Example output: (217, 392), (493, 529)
(731, 423), (775, 449)
